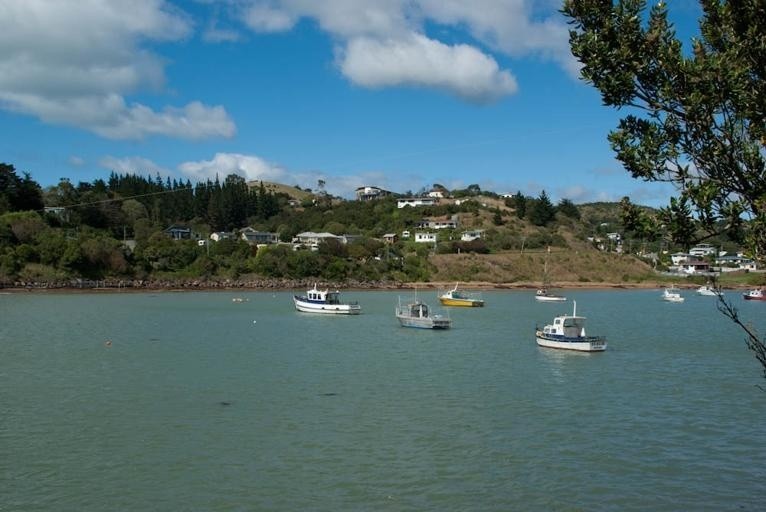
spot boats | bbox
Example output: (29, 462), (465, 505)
(742, 287), (766, 300)
(696, 283), (723, 296)
(292, 282), (361, 315)
(394, 282), (451, 328)
(438, 285), (485, 308)
(661, 283), (684, 303)
(536, 300), (607, 352)
